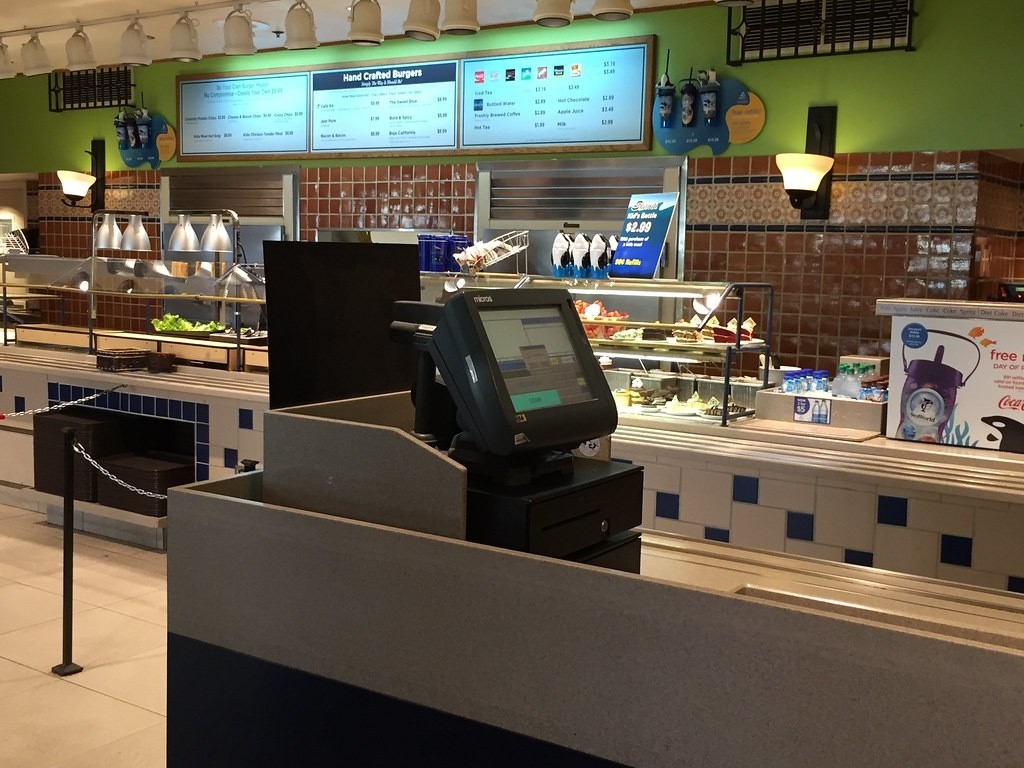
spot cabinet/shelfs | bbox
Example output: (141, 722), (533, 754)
(516, 276), (774, 426)
(0, 249), (267, 371)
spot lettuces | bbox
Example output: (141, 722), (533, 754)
(151, 313), (224, 332)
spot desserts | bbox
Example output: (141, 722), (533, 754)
(573, 299), (753, 417)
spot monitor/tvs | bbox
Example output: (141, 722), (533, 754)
(427, 289), (619, 456)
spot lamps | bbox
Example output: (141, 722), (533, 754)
(21, 33), (52, 77)
(776, 106), (838, 220)
(222, 4), (257, 55)
(167, 12), (202, 63)
(200, 214), (232, 252)
(119, 18), (152, 66)
(590, 0), (634, 21)
(347, 0), (384, 46)
(0, 36), (17, 79)
(95, 213), (123, 250)
(401, 0), (441, 41)
(168, 214), (201, 252)
(532, 0), (575, 28)
(120, 214), (152, 252)
(442, 0), (480, 35)
(66, 25), (98, 72)
(284, 0), (320, 50)
(57, 140), (105, 213)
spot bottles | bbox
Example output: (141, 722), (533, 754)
(812, 399), (827, 424)
(829, 362), (889, 403)
(782, 369), (829, 394)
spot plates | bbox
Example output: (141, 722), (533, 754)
(639, 396), (751, 419)
